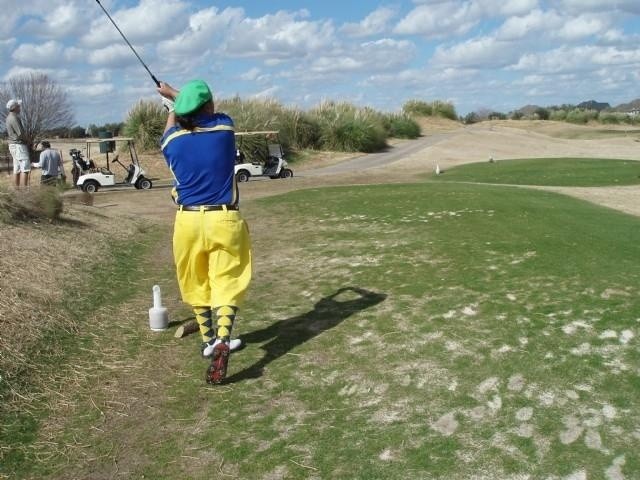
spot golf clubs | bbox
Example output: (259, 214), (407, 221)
(97, 0), (160, 86)
(69, 149), (81, 162)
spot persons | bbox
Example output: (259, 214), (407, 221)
(6, 98), (34, 191)
(156, 79), (253, 386)
(31, 140), (64, 186)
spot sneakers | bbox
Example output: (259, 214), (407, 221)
(201, 337), (242, 357)
(206, 343), (231, 385)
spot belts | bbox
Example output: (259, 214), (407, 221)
(178, 204), (239, 212)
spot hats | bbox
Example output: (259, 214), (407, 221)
(6, 99), (23, 111)
(173, 79), (212, 116)
